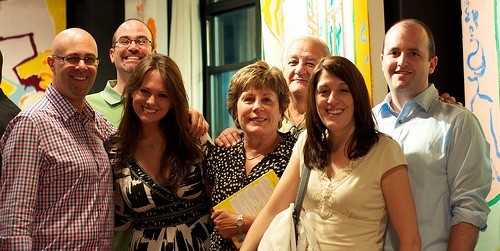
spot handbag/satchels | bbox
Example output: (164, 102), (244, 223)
(257, 163), (305, 251)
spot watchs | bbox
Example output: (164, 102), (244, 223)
(236, 215), (243, 235)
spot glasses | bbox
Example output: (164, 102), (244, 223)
(111, 37), (154, 49)
(52, 54), (100, 66)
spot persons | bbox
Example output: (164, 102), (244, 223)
(102, 55), (215, 251)
(0, 52), (22, 137)
(214, 35), (463, 149)
(361, 18), (492, 251)
(84, 18), (156, 127)
(204, 61), (298, 251)
(0, 28), (209, 251)
(240, 56), (421, 251)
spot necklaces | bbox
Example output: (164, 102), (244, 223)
(245, 151), (267, 160)
